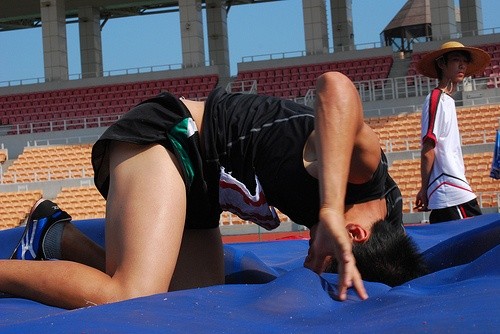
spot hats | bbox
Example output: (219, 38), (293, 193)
(417, 41), (491, 78)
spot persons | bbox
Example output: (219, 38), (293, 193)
(412, 41), (491, 225)
(0, 72), (431, 310)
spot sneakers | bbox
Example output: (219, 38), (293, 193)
(9, 199), (72, 260)
